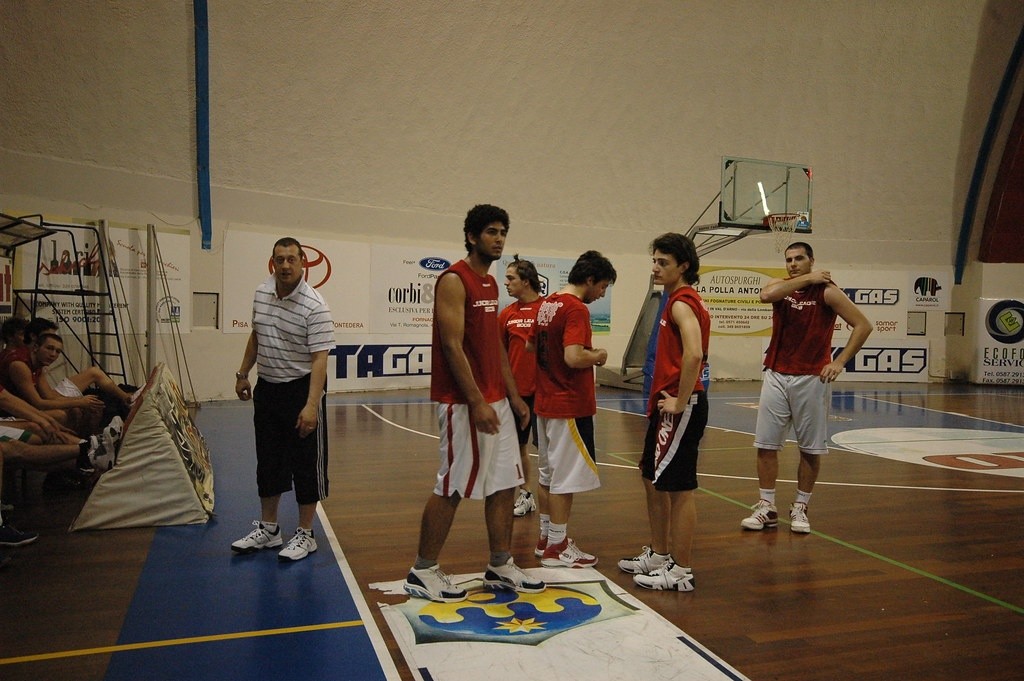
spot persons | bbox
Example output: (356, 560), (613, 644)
(0, 317), (138, 545)
(526, 250), (617, 568)
(230, 237), (336, 561)
(498, 255), (546, 516)
(741, 241), (874, 534)
(402, 204), (546, 604)
(617, 231), (711, 591)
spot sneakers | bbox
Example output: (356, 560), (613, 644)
(741, 499), (779, 529)
(483, 557), (547, 593)
(789, 502), (810, 533)
(542, 536), (598, 568)
(402, 565), (468, 602)
(535, 533), (575, 557)
(231, 520), (283, 550)
(102, 415), (124, 445)
(278, 527), (317, 561)
(129, 383), (147, 409)
(514, 489), (526, 508)
(618, 545), (673, 574)
(0, 520), (39, 546)
(513, 491), (536, 516)
(634, 557), (695, 592)
(87, 432), (114, 470)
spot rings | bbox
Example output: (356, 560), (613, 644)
(309, 427), (313, 429)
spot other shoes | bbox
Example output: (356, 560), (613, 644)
(79, 459), (95, 472)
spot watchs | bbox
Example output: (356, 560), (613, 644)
(236, 372), (247, 379)
